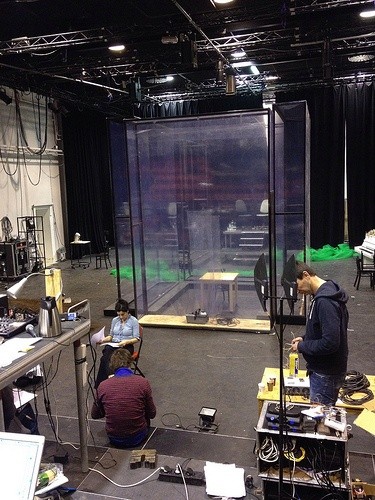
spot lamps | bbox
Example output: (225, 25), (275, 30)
(198, 406), (217, 432)
(8, 268), (62, 314)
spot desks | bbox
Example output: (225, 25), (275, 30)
(199, 271), (239, 312)
(258, 367), (375, 430)
(0, 314), (90, 473)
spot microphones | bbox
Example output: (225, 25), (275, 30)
(25, 324), (37, 337)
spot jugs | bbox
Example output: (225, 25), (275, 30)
(38, 296), (62, 337)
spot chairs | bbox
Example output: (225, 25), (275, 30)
(255, 200), (268, 222)
(96, 240), (112, 269)
(353, 251), (375, 291)
(129, 325), (146, 378)
(167, 202), (177, 226)
(235, 200), (252, 224)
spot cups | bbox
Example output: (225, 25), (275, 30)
(258, 382), (266, 393)
(267, 376), (276, 390)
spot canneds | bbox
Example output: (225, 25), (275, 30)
(289, 352), (299, 375)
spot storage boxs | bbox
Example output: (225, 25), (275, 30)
(0, 240), (31, 280)
(252, 401), (351, 500)
(185, 313), (208, 324)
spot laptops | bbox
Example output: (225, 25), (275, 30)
(0, 432), (46, 500)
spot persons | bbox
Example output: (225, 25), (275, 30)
(91, 348), (156, 449)
(281, 261), (349, 407)
(92, 300), (141, 389)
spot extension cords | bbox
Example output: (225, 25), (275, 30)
(157, 468), (205, 485)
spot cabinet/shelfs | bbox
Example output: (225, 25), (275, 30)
(17, 216), (46, 269)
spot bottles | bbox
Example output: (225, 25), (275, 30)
(289, 351), (299, 375)
